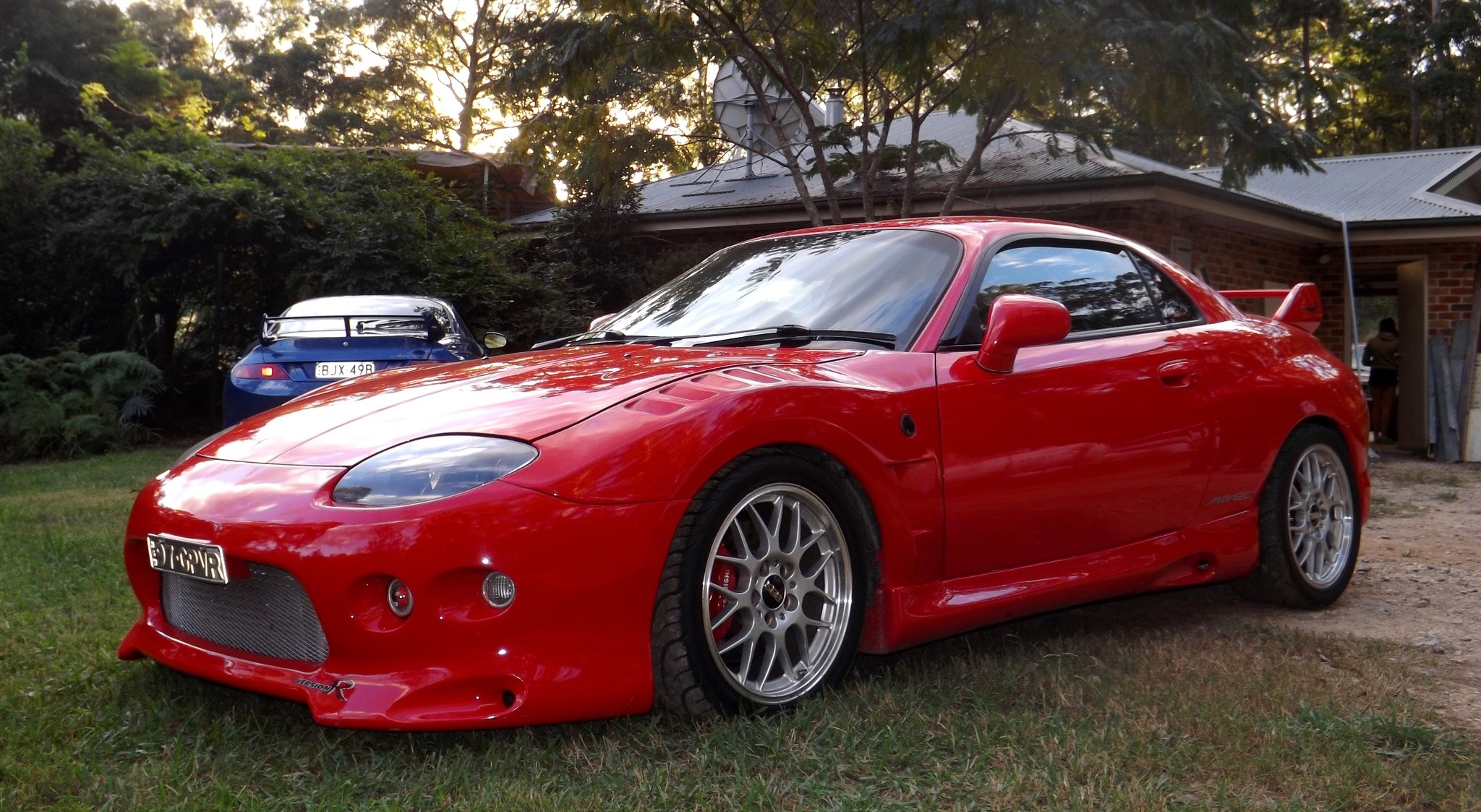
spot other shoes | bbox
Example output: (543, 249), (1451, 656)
(1381, 438), (1393, 443)
(1376, 437), (1381, 442)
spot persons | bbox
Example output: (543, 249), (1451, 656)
(1362, 318), (1400, 443)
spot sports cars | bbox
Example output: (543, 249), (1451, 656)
(114, 212), (1375, 738)
(219, 292), (501, 433)
